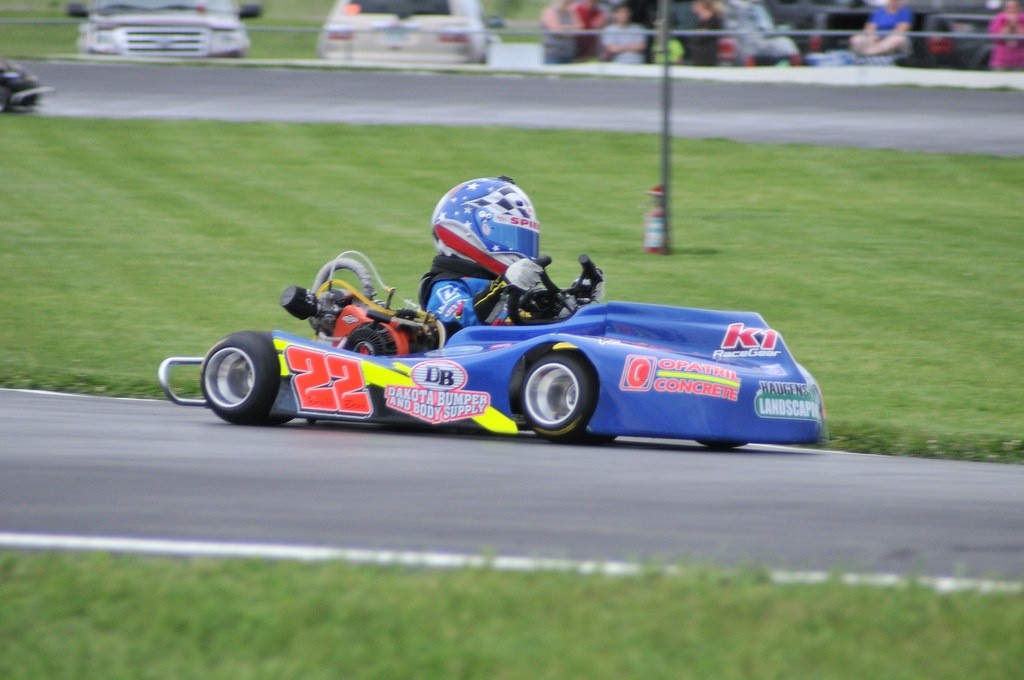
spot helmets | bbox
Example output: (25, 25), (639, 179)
(431, 176), (540, 277)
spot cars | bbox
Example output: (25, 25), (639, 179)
(316, 0), (506, 64)
(67, 0), (264, 60)
(622, 1), (1024, 68)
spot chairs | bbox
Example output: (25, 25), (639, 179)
(419, 276), (457, 350)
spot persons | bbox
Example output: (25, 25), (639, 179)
(984, 0), (1024, 70)
(418, 172), (605, 335)
(676, 0), (724, 67)
(847, 0), (916, 57)
(537, 0), (651, 63)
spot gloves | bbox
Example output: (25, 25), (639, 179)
(571, 267), (606, 304)
(505, 257), (545, 292)
